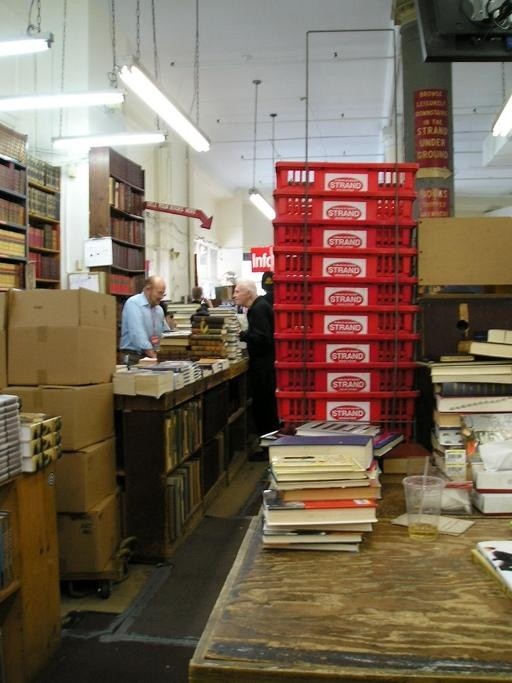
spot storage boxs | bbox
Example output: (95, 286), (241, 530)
(51, 492), (122, 573)
(49, 434), (116, 514)
(271, 160), (418, 446)
(6, 287), (117, 385)
(0, 384), (117, 452)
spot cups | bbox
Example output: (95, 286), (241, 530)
(403, 475), (443, 543)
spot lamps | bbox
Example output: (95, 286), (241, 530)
(0, 2), (56, 55)
(490, 62), (512, 141)
(246, 75), (276, 223)
(50, 2), (170, 151)
(118, 3), (214, 151)
(0, 0), (127, 116)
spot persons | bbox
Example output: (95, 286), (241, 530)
(233, 277), (280, 462)
(261, 272), (274, 305)
(191, 286), (213, 308)
(119, 275), (170, 365)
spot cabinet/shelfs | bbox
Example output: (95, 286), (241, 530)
(115, 355), (250, 565)
(88, 145), (145, 323)
(28, 155), (63, 290)
(0, 153), (28, 291)
(0, 457), (61, 683)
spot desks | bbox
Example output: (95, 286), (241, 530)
(188, 470), (512, 683)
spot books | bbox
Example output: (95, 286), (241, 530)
(28, 223), (60, 250)
(0, 162), (26, 195)
(435, 395), (512, 413)
(109, 177), (144, 216)
(429, 354), (512, 384)
(20, 412), (63, 473)
(164, 461), (202, 545)
(0, 263), (24, 291)
(112, 357), (231, 400)
(156, 304), (201, 361)
(0, 510), (15, 590)
(472, 548), (511, 597)
(431, 432), (470, 489)
(0, 199), (27, 225)
(0, 127), (27, 162)
(261, 420), (407, 552)
(112, 242), (145, 270)
(487, 329), (512, 345)
(111, 217), (145, 245)
(382, 443), (432, 474)
(475, 538), (512, 592)
(434, 410), (512, 430)
(437, 383), (512, 397)
(0, 229), (26, 257)
(0, 395), (23, 483)
(190, 308), (242, 360)
(27, 155), (61, 191)
(165, 400), (203, 470)
(29, 251), (60, 280)
(457, 339), (512, 358)
(111, 275), (145, 293)
(29, 187), (60, 221)
